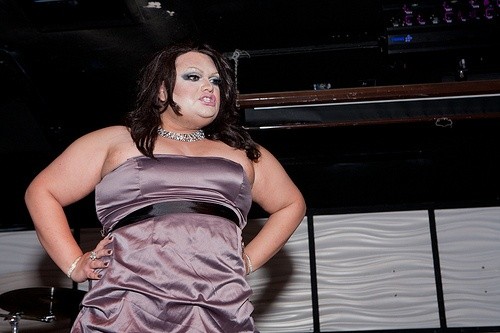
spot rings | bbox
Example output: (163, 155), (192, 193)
(90, 251), (97, 260)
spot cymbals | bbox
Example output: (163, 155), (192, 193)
(1, 288), (89, 324)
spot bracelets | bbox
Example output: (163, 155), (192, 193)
(247, 255), (253, 273)
(67, 257), (81, 278)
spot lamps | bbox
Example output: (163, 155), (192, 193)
(390, 0), (500, 27)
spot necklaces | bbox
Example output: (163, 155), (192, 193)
(158, 127), (205, 142)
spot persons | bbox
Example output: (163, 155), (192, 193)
(25, 45), (306, 333)
